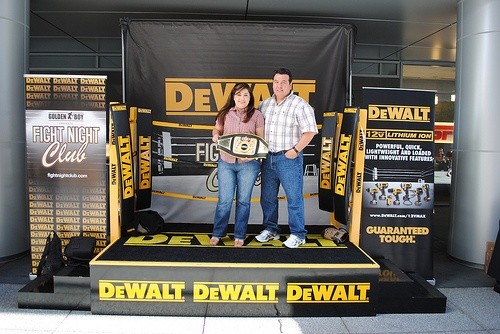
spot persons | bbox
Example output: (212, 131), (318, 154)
(254, 67), (318, 249)
(211, 83), (264, 246)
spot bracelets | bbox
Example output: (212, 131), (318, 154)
(293, 147), (298, 153)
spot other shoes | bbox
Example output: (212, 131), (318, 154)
(235, 239), (245, 246)
(210, 238), (221, 245)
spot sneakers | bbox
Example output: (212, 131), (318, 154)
(282, 234), (306, 248)
(254, 230), (280, 242)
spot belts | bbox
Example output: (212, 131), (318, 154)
(269, 150), (288, 156)
